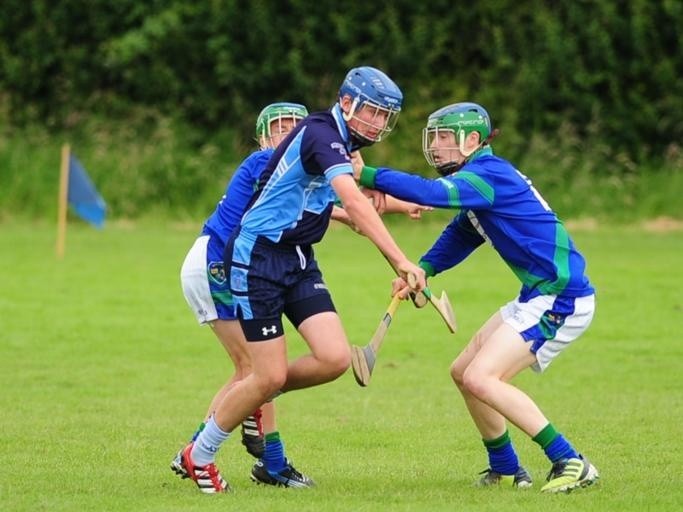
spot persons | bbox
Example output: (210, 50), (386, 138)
(351, 101), (601, 493)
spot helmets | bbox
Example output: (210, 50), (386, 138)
(421, 102), (491, 176)
(256, 102), (308, 151)
(337, 66), (403, 147)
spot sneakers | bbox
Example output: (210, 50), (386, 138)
(168, 409), (316, 493)
(476, 467), (533, 488)
(541, 454), (598, 494)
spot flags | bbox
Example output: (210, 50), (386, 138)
(66, 158), (108, 227)
